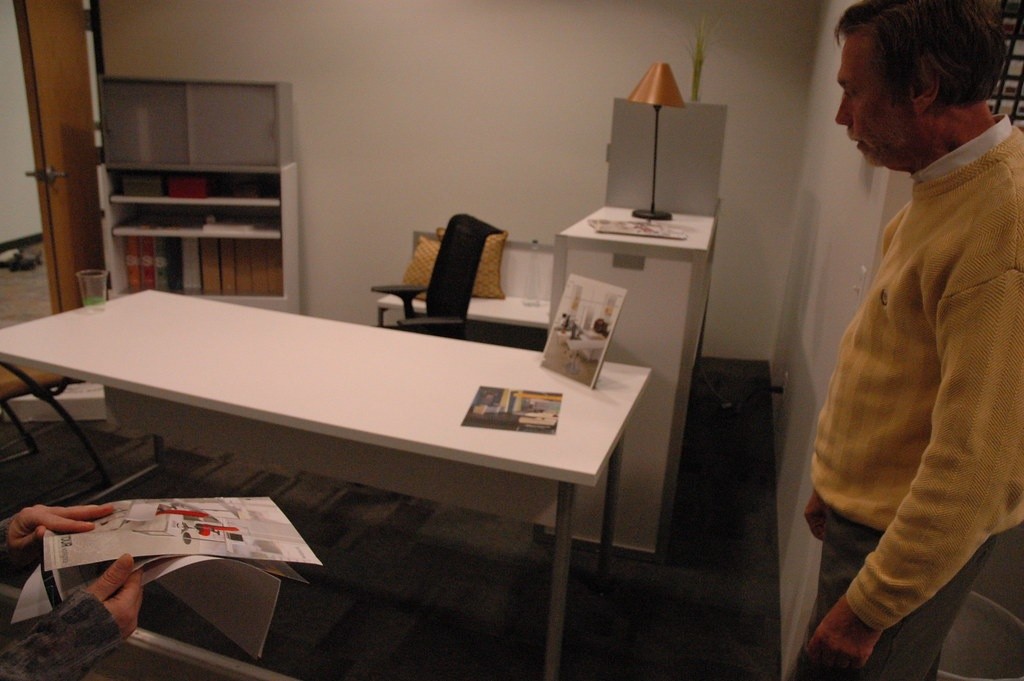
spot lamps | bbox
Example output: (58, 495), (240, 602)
(626, 62), (683, 223)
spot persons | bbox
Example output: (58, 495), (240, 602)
(484, 392), (495, 406)
(0, 504), (144, 681)
(782, 0), (1024, 681)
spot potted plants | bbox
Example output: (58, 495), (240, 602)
(672, 8), (726, 103)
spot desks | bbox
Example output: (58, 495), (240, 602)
(377, 295), (550, 333)
(0, 273), (652, 681)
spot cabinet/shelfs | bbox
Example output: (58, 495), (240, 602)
(530, 99), (729, 560)
(95, 158), (298, 319)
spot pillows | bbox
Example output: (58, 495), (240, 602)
(403, 230), (511, 299)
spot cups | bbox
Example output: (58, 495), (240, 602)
(76, 269), (108, 313)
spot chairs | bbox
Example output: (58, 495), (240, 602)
(370, 214), (498, 339)
(1, 361), (166, 523)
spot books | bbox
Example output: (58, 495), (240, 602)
(123, 238), (284, 297)
(588, 219), (688, 239)
(540, 272), (627, 389)
